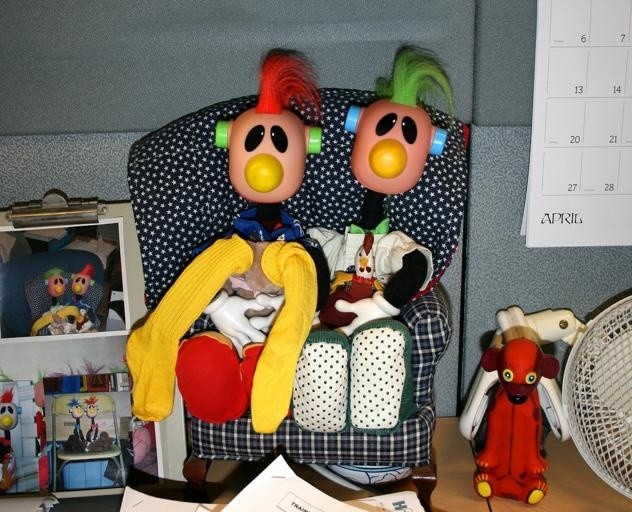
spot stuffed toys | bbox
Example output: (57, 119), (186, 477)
(62, 264), (105, 333)
(290, 46), (459, 439)
(41, 267), (74, 335)
(85, 395), (99, 451)
(124, 47), (326, 436)
(67, 399), (88, 452)
(0, 386), (21, 491)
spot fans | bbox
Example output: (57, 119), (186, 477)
(562, 294), (632, 500)
